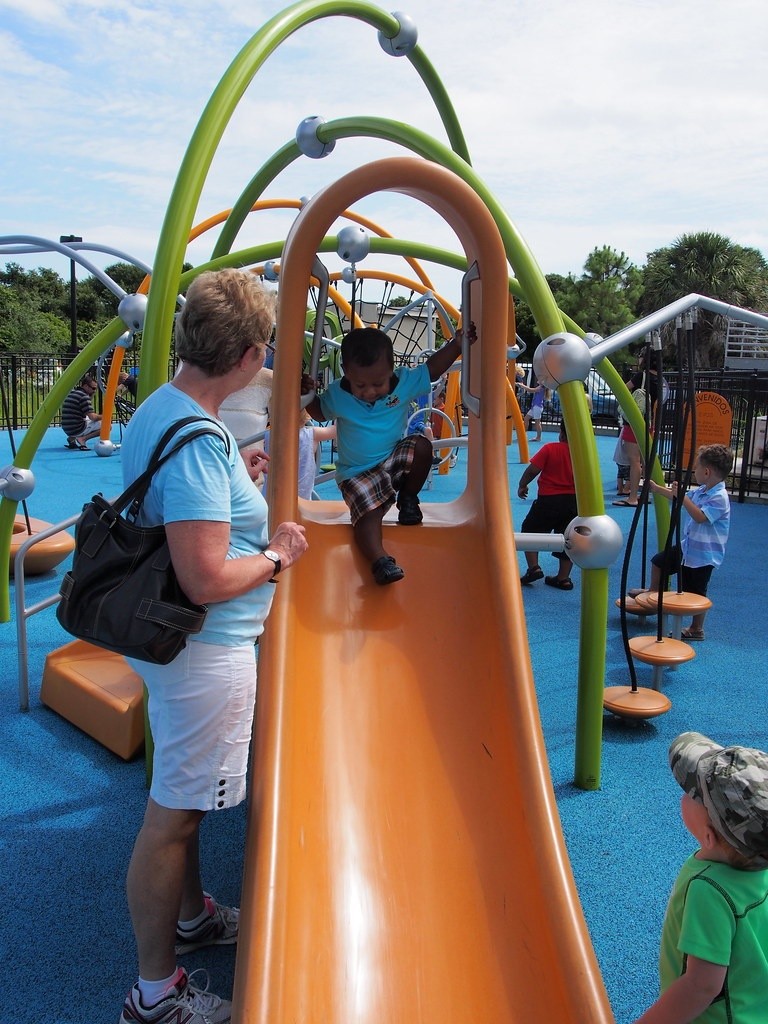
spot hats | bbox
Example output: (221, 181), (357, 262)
(669, 732), (768, 864)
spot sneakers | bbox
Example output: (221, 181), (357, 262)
(396, 491), (423, 524)
(119, 968), (232, 1024)
(372, 556), (404, 586)
(172, 892), (243, 955)
(629, 588), (650, 600)
(668, 627), (705, 640)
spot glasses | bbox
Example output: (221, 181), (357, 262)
(265, 343), (276, 357)
(88, 384), (97, 391)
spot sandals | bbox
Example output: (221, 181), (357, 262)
(545, 575), (573, 590)
(63, 437), (91, 451)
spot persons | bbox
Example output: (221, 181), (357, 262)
(61, 377), (112, 451)
(301, 319), (478, 586)
(517, 417), (580, 590)
(115, 269), (309, 1024)
(636, 731), (768, 1024)
(612, 348), (659, 507)
(628, 444), (734, 641)
(514, 380), (552, 441)
(430, 390), (458, 468)
(116, 372), (138, 401)
(218, 367), (337, 500)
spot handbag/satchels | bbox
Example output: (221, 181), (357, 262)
(57, 414), (230, 666)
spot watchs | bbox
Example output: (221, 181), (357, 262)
(260, 550), (281, 583)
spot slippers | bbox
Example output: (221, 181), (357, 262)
(617, 490), (631, 496)
(611, 496), (651, 507)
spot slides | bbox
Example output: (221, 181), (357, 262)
(229, 502), (617, 1024)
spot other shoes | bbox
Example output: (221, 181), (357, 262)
(449, 454), (458, 468)
(432, 456), (442, 465)
(529, 437), (540, 442)
(521, 566), (543, 584)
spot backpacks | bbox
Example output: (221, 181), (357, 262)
(618, 371), (651, 424)
